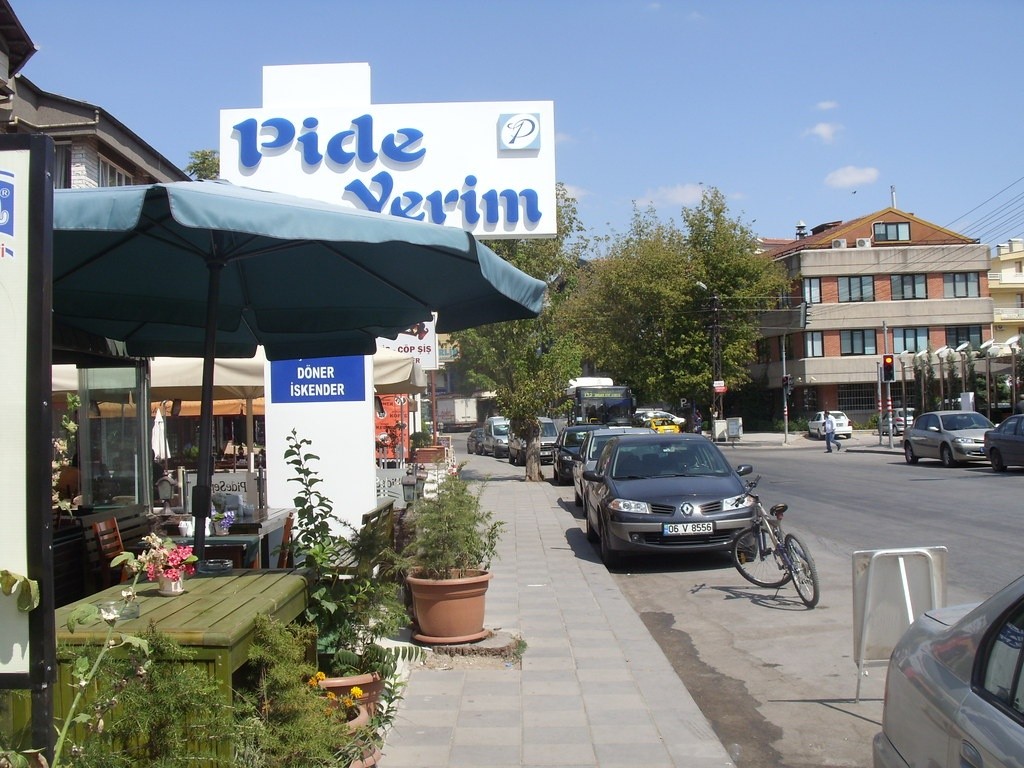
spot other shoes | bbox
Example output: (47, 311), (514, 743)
(824, 450), (831, 453)
(837, 444), (841, 450)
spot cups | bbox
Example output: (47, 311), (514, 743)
(178, 521), (192, 537)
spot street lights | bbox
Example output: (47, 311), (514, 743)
(934, 346), (948, 410)
(980, 338), (995, 421)
(916, 349), (928, 413)
(954, 341), (970, 392)
(1005, 335), (1020, 414)
(696, 281), (724, 420)
(896, 349), (909, 429)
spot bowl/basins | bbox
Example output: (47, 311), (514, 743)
(198, 559), (233, 573)
(101, 601), (140, 620)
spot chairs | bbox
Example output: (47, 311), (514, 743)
(278, 511), (295, 568)
(91, 518), (132, 590)
(675, 449), (699, 471)
(616, 455), (642, 477)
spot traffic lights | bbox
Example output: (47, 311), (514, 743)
(800, 301), (813, 329)
(783, 375), (788, 394)
(882, 354), (896, 383)
(788, 374), (794, 396)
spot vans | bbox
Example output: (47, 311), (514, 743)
(508, 416), (559, 466)
(483, 416), (510, 458)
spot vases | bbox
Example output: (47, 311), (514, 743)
(154, 572), (185, 597)
(311, 698), (382, 768)
(213, 521), (230, 536)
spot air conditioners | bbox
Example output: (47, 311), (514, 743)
(996, 325), (1004, 331)
(856, 238), (872, 248)
(831, 238), (846, 248)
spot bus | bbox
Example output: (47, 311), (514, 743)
(551, 377), (637, 426)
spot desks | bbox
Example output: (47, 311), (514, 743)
(208, 507), (297, 567)
(137, 530), (264, 570)
(51, 566), (318, 768)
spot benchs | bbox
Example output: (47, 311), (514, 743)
(312, 497), (395, 582)
(80, 502), (152, 573)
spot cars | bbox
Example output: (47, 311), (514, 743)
(877, 408), (914, 436)
(582, 433), (760, 571)
(552, 425), (658, 517)
(872, 574), (1024, 768)
(642, 418), (683, 434)
(807, 410), (853, 440)
(634, 411), (686, 426)
(467, 428), (484, 455)
(982, 414), (1024, 472)
(903, 410), (997, 468)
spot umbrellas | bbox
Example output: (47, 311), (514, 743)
(51, 177), (549, 563)
(151, 408), (171, 469)
(51, 342), (426, 474)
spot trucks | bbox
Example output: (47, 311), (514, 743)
(436, 398), (492, 433)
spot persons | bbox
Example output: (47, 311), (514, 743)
(824, 411), (841, 453)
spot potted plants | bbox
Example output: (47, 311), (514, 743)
(286, 430), (426, 718)
(381, 454), (509, 645)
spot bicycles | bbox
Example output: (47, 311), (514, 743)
(731, 474), (820, 608)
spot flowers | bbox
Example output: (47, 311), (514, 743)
(124, 533), (199, 581)
(306, 671), (363, 724)
(211, 509), (235, 531)
